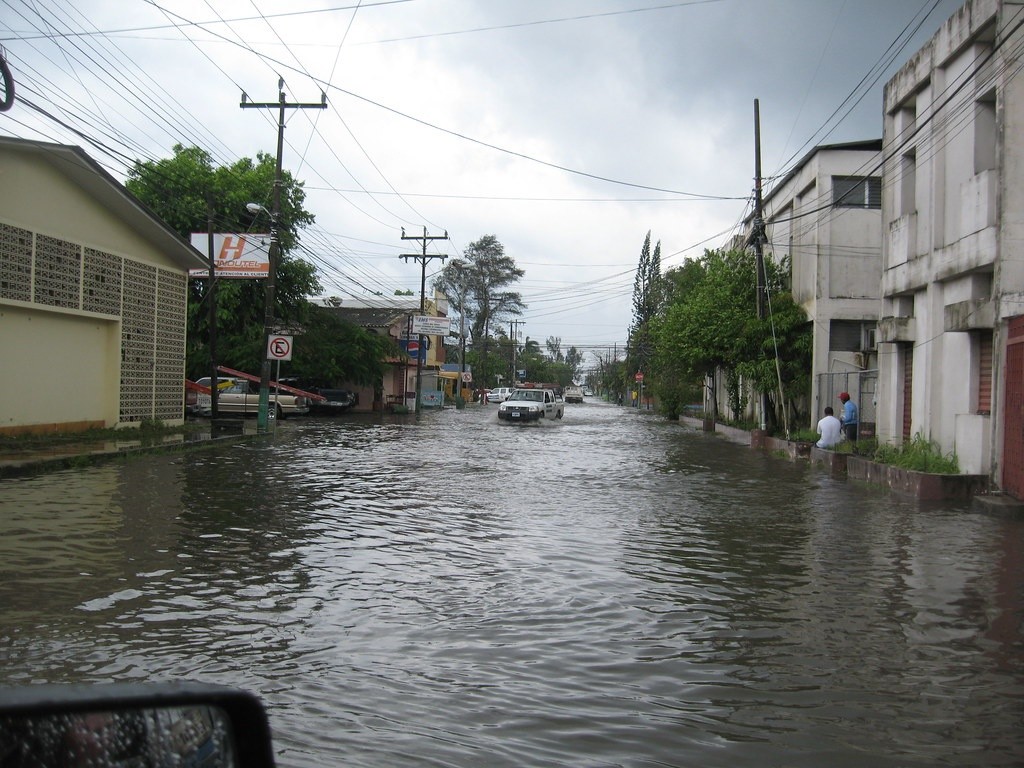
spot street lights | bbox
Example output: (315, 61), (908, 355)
(244, 202), (278, 432)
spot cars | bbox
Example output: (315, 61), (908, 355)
(478, 387), (519, 403)
(584, 389), (592, 397)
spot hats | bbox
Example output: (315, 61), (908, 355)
(837, 392), (850, 400)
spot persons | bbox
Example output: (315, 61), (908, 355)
(839, 393), (857, 441)
(618, 391), (623, 406)
(473, 391), (478, 402)
(631, 389), (637, 407)
(815, 407), (841, 448)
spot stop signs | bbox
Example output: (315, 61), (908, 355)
(635, 373), (643, 382)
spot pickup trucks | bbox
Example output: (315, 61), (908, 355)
(268, 378), (359, 415)
(497, 388), (565, 423)
(191, 377), (311, 420)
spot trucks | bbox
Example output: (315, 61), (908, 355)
(564, 386), (584, 402)
